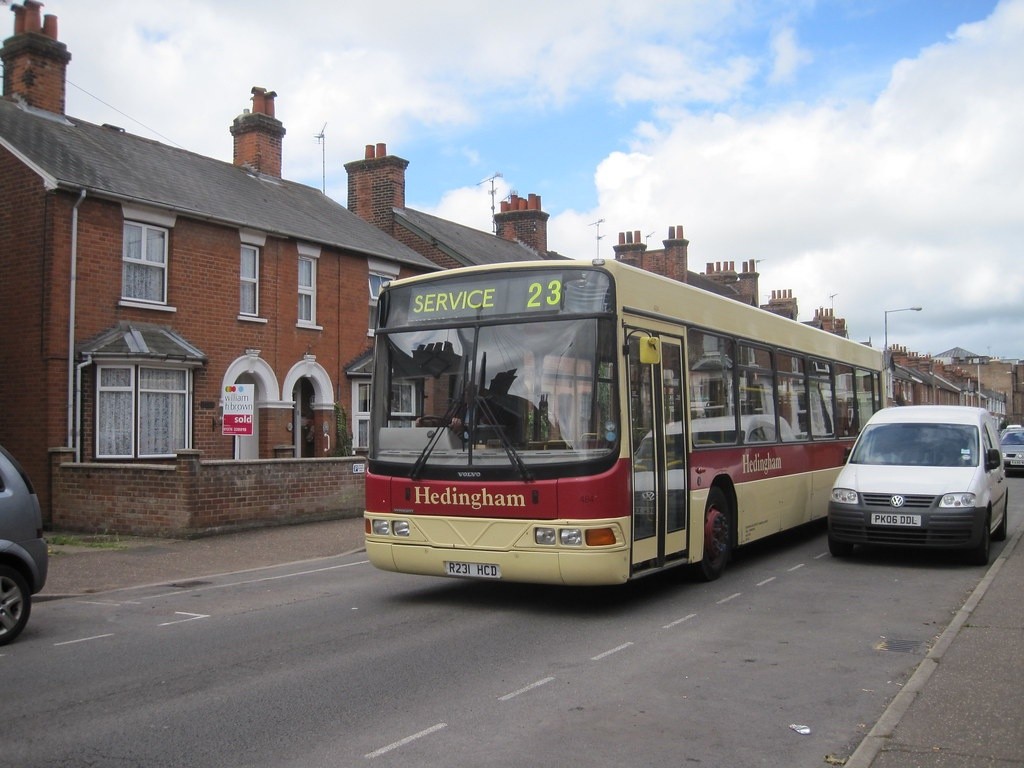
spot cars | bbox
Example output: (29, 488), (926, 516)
(1000, 424), (1024, 476)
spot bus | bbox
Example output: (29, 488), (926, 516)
(363, 257), (893, 586)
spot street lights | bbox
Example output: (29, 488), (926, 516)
(885, 307), (923, 405)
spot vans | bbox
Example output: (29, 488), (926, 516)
(827, 405), (1008, 565)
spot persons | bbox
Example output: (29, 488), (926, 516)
(863, 430), (917, 465)
(416, 373), (507, 443)
